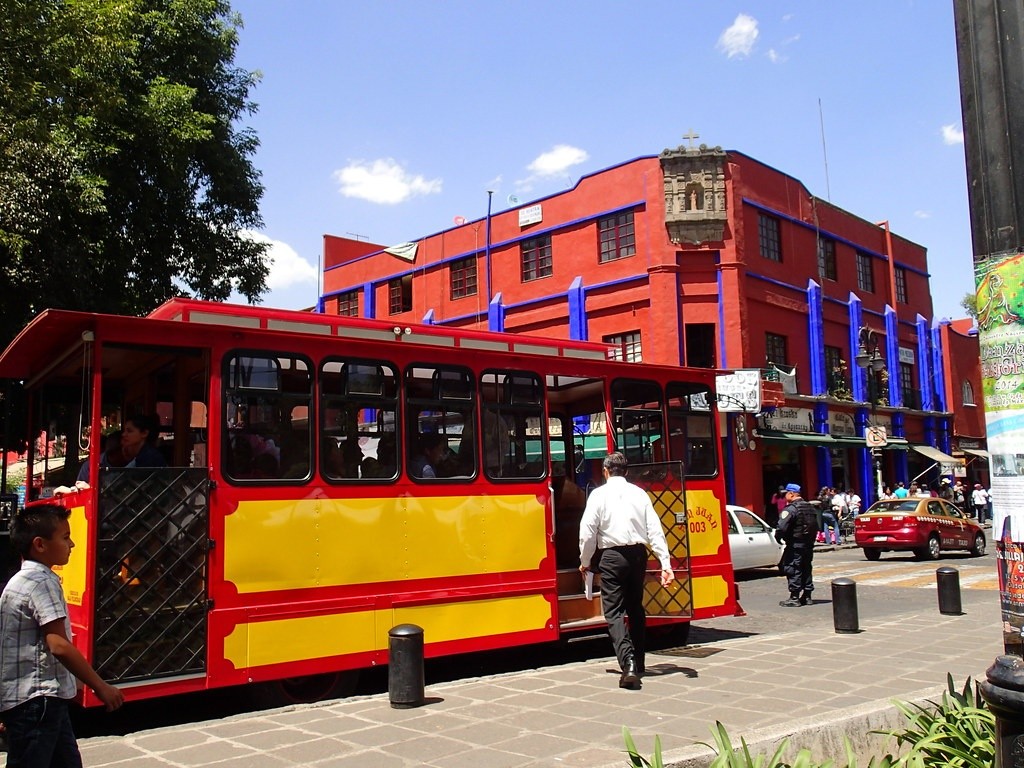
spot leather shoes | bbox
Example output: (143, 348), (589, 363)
(633, 659), (645, 674)
(619, 660), (638, 688)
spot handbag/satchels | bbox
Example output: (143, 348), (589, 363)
(822, 512), (833, 519)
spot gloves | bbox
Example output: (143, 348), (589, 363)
(775, 536), (783, 545)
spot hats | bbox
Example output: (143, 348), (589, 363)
(781, 484), (801, 493)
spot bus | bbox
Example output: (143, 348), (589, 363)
(0, 295), (746, 737)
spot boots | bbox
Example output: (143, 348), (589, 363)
(780, 592), (802, 607)
(799, 591), (813, 605)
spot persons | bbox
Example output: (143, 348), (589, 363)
(818, 486), (862, 545)
(771, 485), (789, 514)
(776, 483), (819, 607)
(579, 451), (674, 688)
(0, 504), (125, 768)
(460, 394), (508, 479)
(77, 409), (176, 488)
(320, 430), (450, 480)
(881, 478), (993, 526)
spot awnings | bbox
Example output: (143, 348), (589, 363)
(959, 449), (989, 467)
(752, 429), (909, 450)
(904, 445), (960, 488)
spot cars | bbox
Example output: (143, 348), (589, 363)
(725, 506), (787, 572)
(855, 496), (986, 560)
